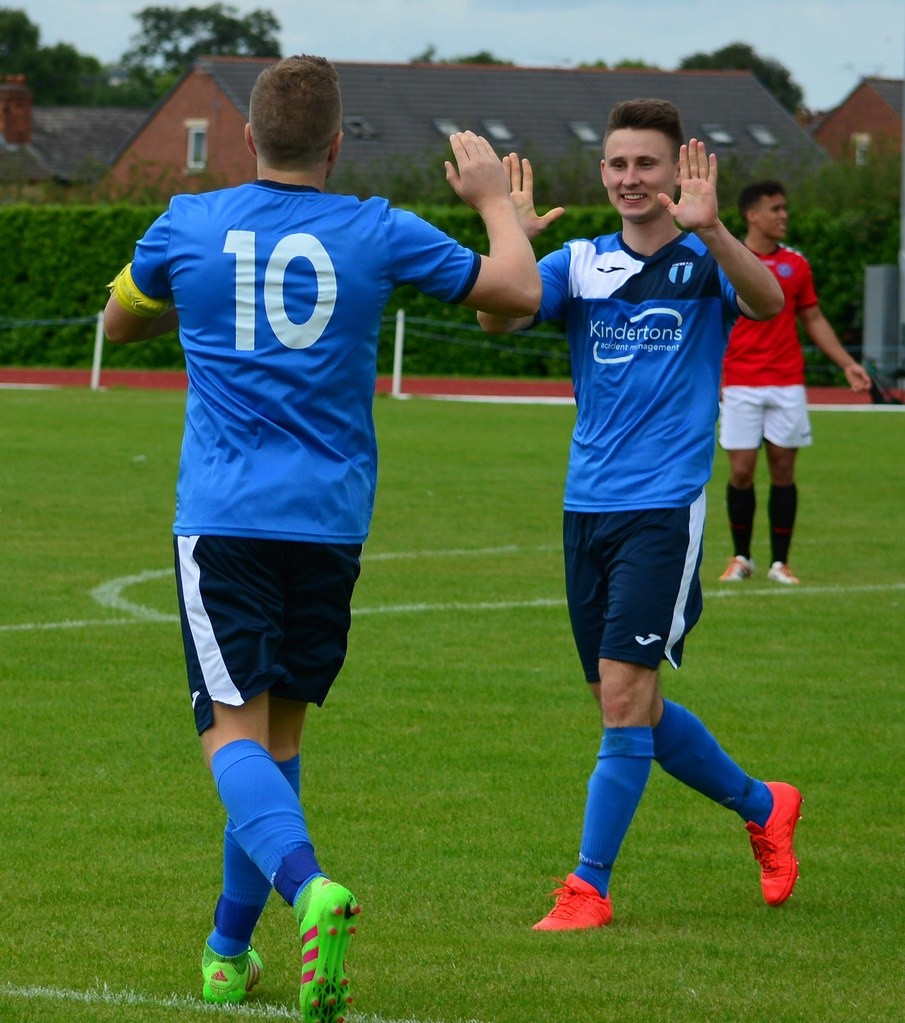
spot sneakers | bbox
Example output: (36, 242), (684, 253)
(717, 554), (753, 581)
(531, 872), (614, 932)
(293, 875), (360, 1023)
(764, 560), (800, 586)
(745, 779), (806, 909)
(199, 937), (264, 1008)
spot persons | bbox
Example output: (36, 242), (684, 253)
(715, 181), (871, 582)
(478, 102), (802, 932)
(103, 52), (542, 1023)
